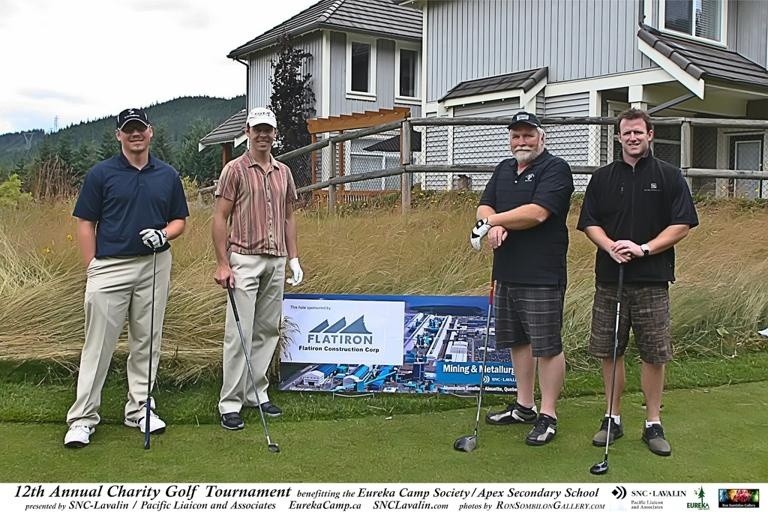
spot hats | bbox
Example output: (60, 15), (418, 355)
(507, 112), (542, 129)
(246, 108), (277, 129)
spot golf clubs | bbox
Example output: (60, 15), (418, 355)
(225, 279), (281, 452)
(588, 264), (626, 475)
(453, 246), (500, 452)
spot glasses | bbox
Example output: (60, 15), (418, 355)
(116, 108), (152, 131)
(123, 124), (146, 132)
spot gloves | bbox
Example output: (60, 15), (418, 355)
(139, 229), (169, 248)
(470, 217), (491, 251)
(287, 258), (304, 286)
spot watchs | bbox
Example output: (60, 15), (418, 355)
(640, 243), (650, 258)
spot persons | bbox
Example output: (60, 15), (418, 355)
(63, 108), (190, 449)
(213, 107), (304, 431)
(469, 111), (575, 446)
(576, 108), (700, 456)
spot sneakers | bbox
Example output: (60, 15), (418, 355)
(526, 414), (557, 445)
(258, 402), (282, 416)
(642, 419), (672, 456)
(486, 400), (536, 424)
(63, 421), (95, 448)
(123, 407), (166, 435)
(219, 413), (244, 430)
(592, 418), (624, 446)
